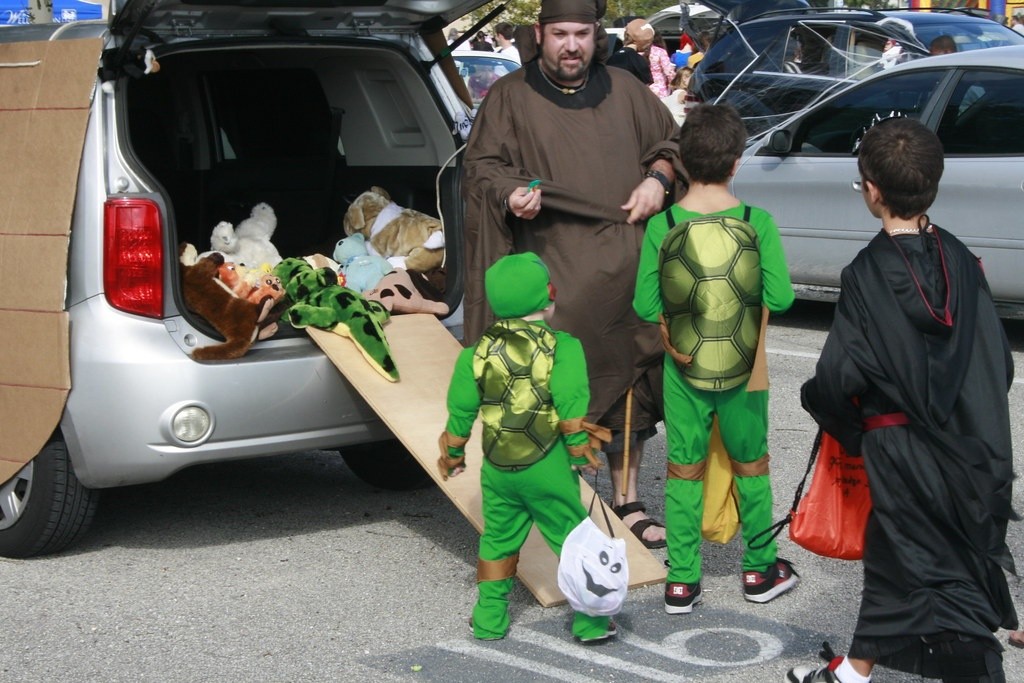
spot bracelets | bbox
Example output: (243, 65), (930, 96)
(645, 170), (671, 195)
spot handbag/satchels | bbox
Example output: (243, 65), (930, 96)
(746, 423), (871, 562)
(557, 465), (629, 618)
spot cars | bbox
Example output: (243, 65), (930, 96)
(728, 43), (1023, 321)
(449, 50), (523, 111)
(0, 1), (514, 561)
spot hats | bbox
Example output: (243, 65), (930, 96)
(625, 19), (655, 51)
(485, 252), (552, 319)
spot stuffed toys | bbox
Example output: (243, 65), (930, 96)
(174, 185), (450, 382)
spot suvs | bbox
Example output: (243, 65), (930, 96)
(605, 1), (1024, 139)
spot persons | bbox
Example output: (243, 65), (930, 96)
(446, 21), (539, 99)
(459, 0), (690, 549)
(930, 35), (957, 56)
(437, 250), (617, 641)
(1010, 15), (1024, 36)
(606, 18), (705, 129)
(783, 117), (1024, 683)
(633, 106), (800, 615)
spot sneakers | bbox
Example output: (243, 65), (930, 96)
(743, 556), (801, 603)
(664, 581), (702, 614)
(783, 640), (873, 683)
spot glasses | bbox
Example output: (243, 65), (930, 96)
(852, 175), (876, 192)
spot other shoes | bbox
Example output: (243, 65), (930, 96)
(469, 617), (474, 632)
(580, 618), (618, 641)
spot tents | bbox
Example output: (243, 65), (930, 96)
(0, 0), (102, 28)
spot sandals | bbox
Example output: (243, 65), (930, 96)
(610, 500), (669, 549)
(1009, 638), (1024, 648)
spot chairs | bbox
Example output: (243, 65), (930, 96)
(212, 65), (339, 251)
(853, 42), (886, 81)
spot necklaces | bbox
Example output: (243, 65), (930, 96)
(538, 62), (589, 94)
(889, 228), (919, 233)
